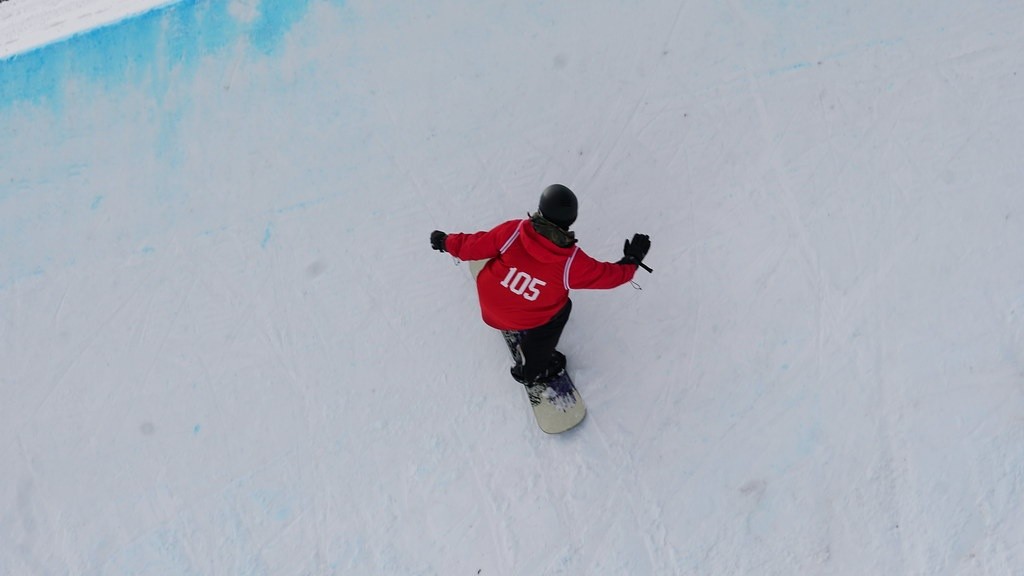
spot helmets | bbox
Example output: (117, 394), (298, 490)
(539, 184), (578, 226)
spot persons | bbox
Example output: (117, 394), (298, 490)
(430, 184), (651, 389)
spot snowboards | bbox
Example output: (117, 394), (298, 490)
(467, 232), (587, 436)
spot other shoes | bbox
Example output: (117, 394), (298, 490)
(511, 350), (565, 384)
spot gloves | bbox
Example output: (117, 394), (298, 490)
(623, 233), (650, 263)
(431, 231), (449, 253)
